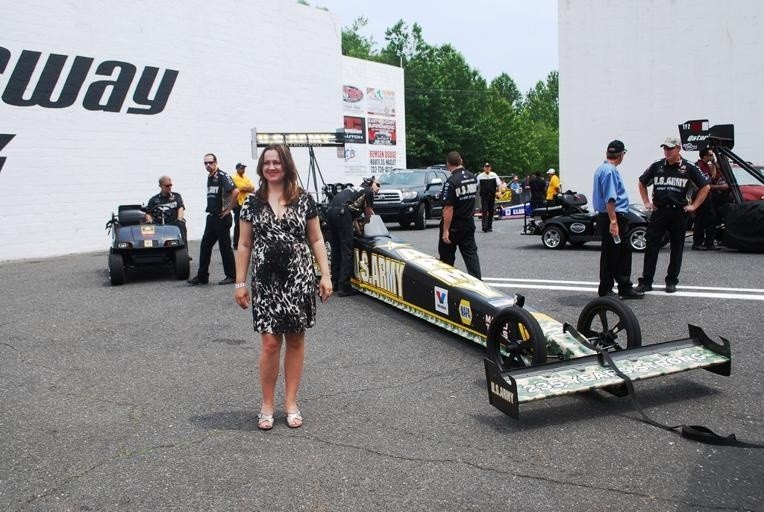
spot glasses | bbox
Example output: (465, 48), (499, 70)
(205, 162), (215, 164)
(163, 184), (172, 187)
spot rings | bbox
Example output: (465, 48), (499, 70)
(330, 290), (333, 293)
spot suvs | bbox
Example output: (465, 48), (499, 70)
(363, 158), (452, 230)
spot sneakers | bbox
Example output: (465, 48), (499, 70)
(482, 227), (492, 232)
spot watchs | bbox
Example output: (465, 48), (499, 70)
(611, 220), (617, 225)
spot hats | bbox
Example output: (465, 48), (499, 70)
(236, 163), (246, 169)
(660, 138), (680, 148)
(485, 163), (490, 166)
(546, 168), (556, 175)
(607, 140), (627, 153)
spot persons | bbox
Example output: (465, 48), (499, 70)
(145, 176), (192, 261)
(477, 163), (501, 233)
(328, 178), (380, 298)
(635, 139), (711, 294)
(231, 163), (256, 250)
(592, 140), (645, 301)
(187, 154), (239, 285)
(438, 152), (481, 283)
(235, 145), (333, 431)
(498, 172), (546, 216)
(546, 168), (562, 207)
(691, 147), (728, 251)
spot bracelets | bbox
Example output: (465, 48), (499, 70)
(236, 283), (245, 288)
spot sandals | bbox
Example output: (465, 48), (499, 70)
(257, 414), (274, 429)
(284, 411), (302, 428)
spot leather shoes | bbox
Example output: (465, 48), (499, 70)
(219, 276), (236, 285)
(709, 246), (721, 250)
(692, 244), (708, 251)
(337, 290), (357, 297)
(636, 284), (652, 292)
(600, 291), (623, 298)
(618, 289), (644, 300)
(187, 276), (209, 284)
(666, 283), (676, 293)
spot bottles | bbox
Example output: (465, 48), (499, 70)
(610, 227), (623, 245)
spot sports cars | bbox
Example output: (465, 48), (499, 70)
(491, 201), (531, 218)
(246, 125), (738, 425)
(676, 116), (764, 255)
